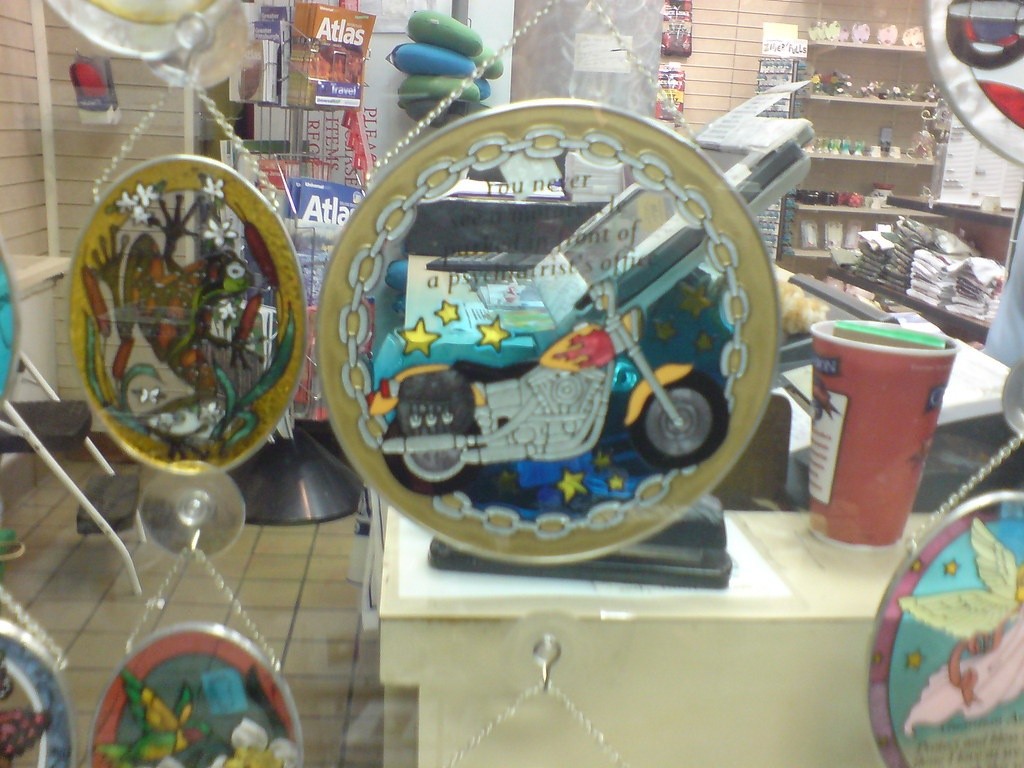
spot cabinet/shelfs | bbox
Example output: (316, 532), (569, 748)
(827, 194), (1014, 340)
(780, 41), (950, 261)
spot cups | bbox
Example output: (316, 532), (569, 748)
(810, 321), (959, 551)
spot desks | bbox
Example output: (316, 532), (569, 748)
(380, 508), (955, 768)
(0, 253), (67, 404)
